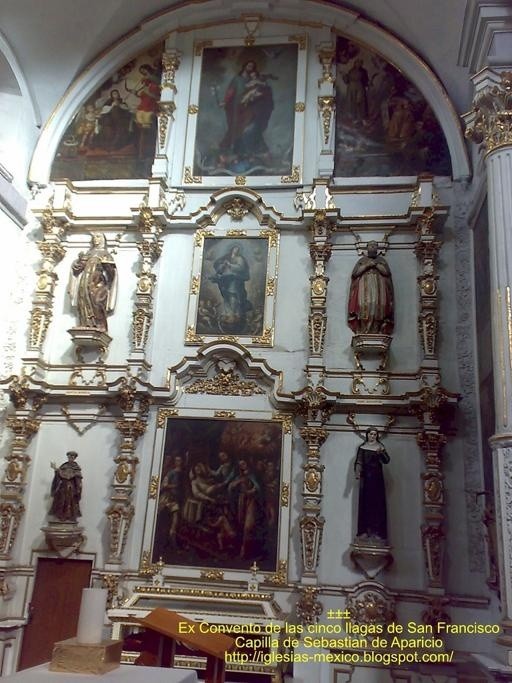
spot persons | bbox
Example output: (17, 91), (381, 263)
(160, 449), (279, 561)
(48, 451), (83, 521)
(79, 64), (162, 151)
(219, 60), (274, 160)
(354, 426), (390, 546)
(348, 240), (395, 334)
(213, 246), (253, 314)
(339, 58), (444, 151)
(72, 232), (116, 332)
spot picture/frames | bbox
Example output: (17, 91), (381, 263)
(183, 225), (280, 349)
(180, 31), (308, 190)
(136, 405), (294, 588)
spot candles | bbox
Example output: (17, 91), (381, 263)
(75, 586), (109, 645)
